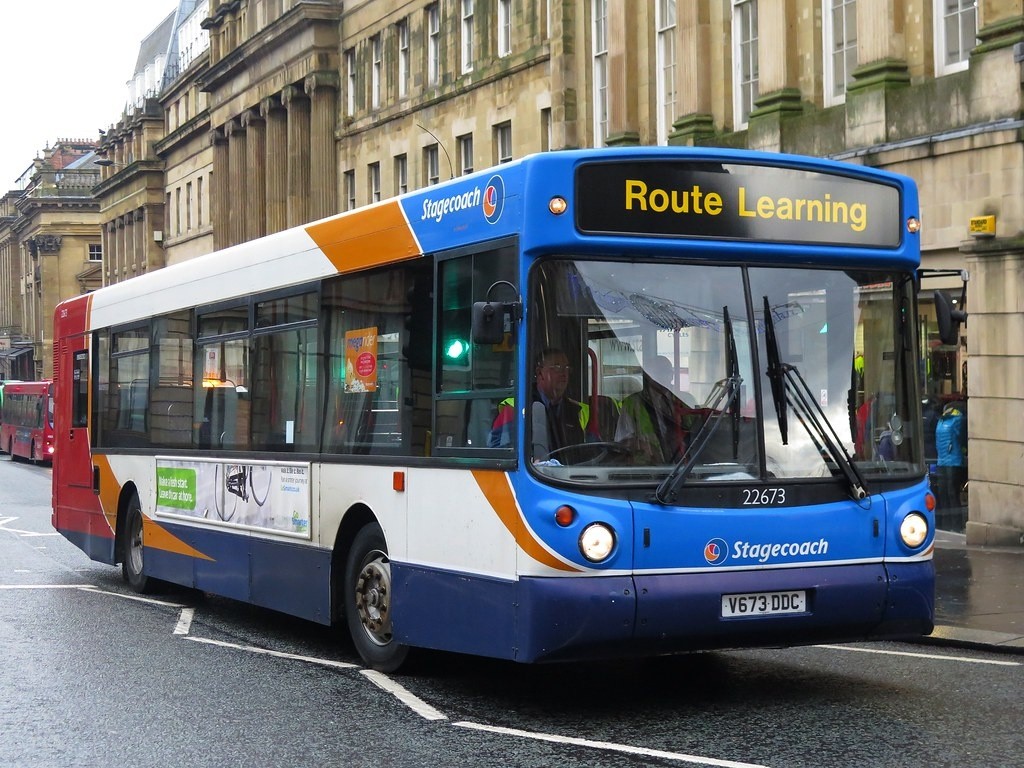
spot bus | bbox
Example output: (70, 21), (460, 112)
(48, 145), (969, 678)
(0, 379), (55, 467)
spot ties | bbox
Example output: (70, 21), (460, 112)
(661, 395), (674, 430)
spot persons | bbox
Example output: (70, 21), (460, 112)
(920, 396), (944, 529)
(875, 411), (899, 462)
(612, 356), (698, 463)
(934, 408), (967, 533)
(487, 348), (620, 465)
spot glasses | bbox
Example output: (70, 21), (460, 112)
(539, 364), (570, 373)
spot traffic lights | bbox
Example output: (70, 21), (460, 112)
(441, 336), (471, 364)
